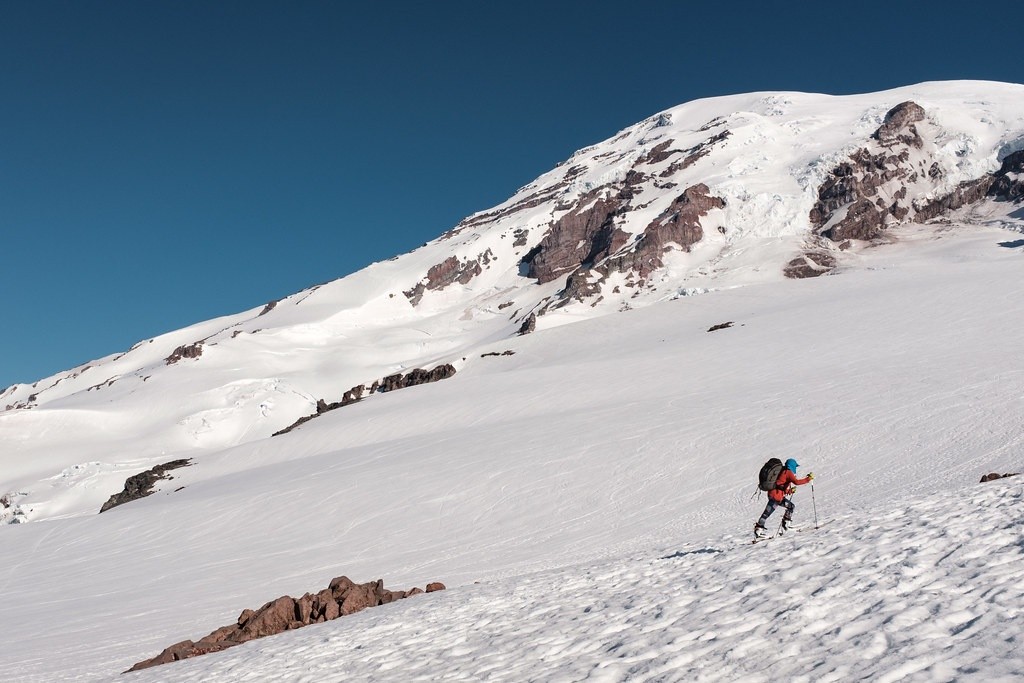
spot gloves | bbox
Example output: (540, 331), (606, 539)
(791, 486), (797, 493)
(807, 471), (815, 479)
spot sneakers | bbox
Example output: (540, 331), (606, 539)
(782, 517), (791, 531)
(754, 523), (764, 537)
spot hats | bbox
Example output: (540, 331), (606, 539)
(785, 458), (800, 474)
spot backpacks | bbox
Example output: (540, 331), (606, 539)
(758, 458), (790, 490)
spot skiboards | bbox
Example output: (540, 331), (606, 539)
(752, 518), (835, 545)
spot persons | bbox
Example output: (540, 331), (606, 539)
(753, 458), (815, 538)
